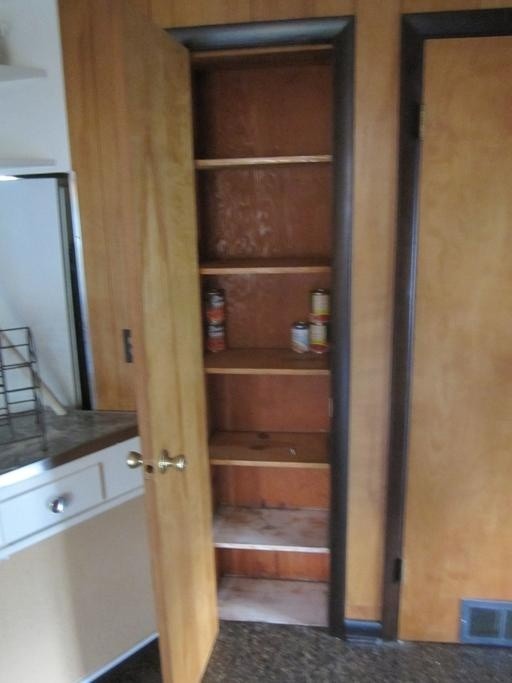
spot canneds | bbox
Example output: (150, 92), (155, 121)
(309, 288), (330, 323)
(291, 323), (309, 353)
(309, 323), (329, 353)
(205, 324), (227, 353)
(204, 289), (225, 324)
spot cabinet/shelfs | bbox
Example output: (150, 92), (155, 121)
(187, 34), (338, 582)
